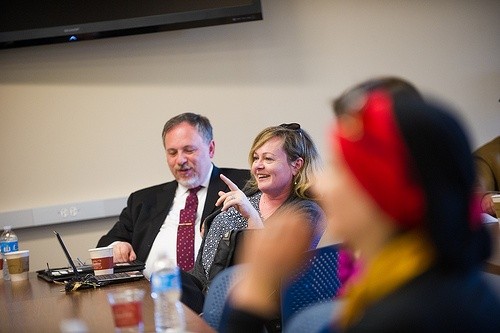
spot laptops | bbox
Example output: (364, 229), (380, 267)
(53, 230), (145, 288)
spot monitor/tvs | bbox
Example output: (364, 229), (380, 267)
(0, 0), (264, 51)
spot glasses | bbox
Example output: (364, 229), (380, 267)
(279, 123), (303, 138)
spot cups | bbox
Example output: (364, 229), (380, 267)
(87, 247), (114, 277)
(107, 289), (145, 332)
(4, 250), (31, 281)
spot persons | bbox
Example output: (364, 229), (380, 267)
(216, 78), (500, 333)
(191, 122), (326, 294)
(96, 112), (251, 282)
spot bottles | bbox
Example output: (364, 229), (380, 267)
(0, 225), (18, 280)
(149, 252), (185, 333)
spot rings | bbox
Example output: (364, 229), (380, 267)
(230, 195), (235, 199)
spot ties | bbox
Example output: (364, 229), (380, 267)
(177, 186), (204, 277)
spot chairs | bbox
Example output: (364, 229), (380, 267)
(279, 244), (342, 333)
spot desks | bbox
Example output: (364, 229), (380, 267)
(0, 271), (217, 333)
